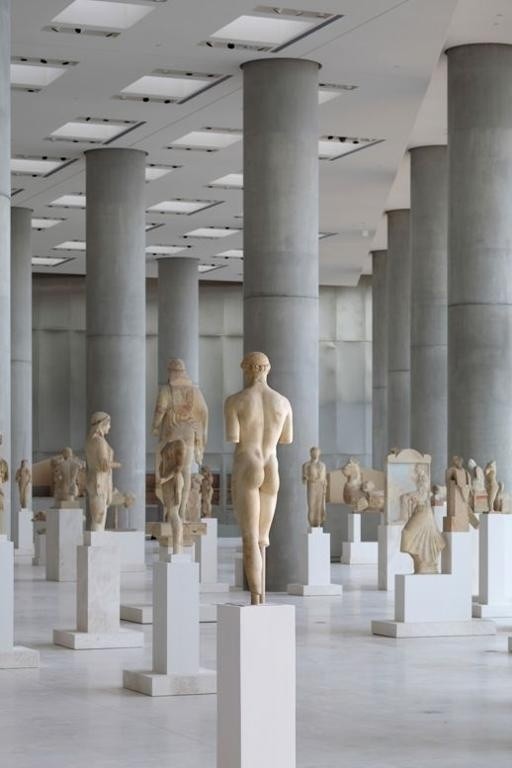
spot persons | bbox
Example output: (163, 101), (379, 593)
(304, 445), (503, 576)
(0, 441), (214, 548)
(150, 356), (210, 557)
(220, 347), (293, 604)
(82, 410), (124, 532)
